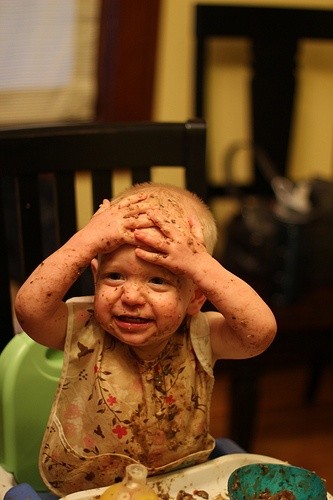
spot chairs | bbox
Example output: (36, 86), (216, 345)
(0, 120), (333, 453)
(0, 333), (241, 500)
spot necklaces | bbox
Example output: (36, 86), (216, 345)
(125, 334), (177, 387)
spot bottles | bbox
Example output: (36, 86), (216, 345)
(101, 464), (158, 500)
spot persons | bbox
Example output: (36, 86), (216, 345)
(13, 183), (278, 498)
